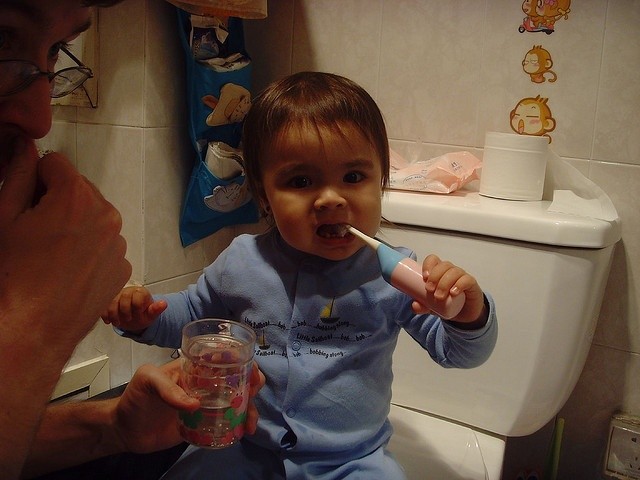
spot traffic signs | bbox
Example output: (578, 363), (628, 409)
(600, 412), (640, 480)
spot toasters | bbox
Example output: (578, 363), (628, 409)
(337, 226), (471, 319)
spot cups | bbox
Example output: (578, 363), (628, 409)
(0, 47), (93, 99)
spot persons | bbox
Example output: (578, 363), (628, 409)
(0, 0), (267, 480)
(101, 71), (497, 480)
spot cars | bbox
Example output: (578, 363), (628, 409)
(178, 318), (257, 447)
